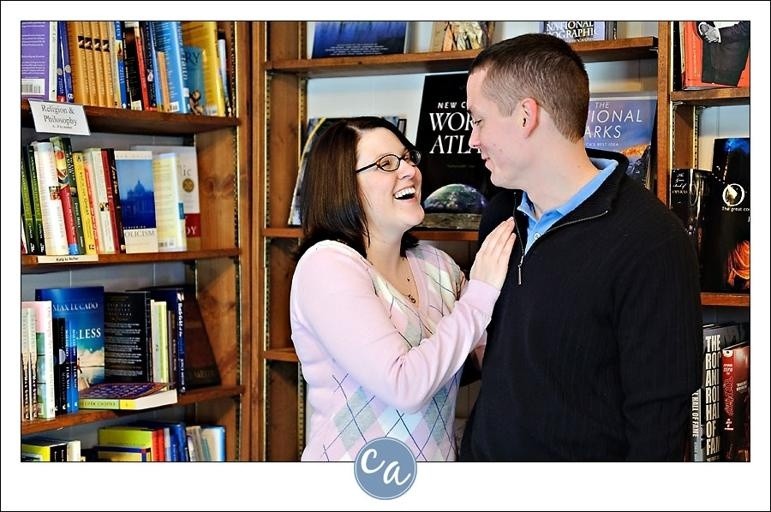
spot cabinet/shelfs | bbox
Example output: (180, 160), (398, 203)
(21, 22), (252, 460)
(253, 19), (671, 462)
(672, 21), (752, 460)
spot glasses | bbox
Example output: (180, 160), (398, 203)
(356, 150), (422, 174)
